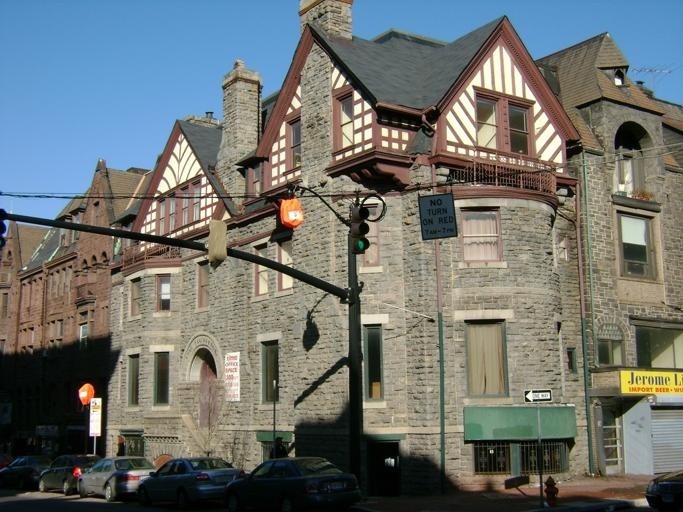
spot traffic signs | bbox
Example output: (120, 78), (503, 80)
(523, 388), (552, 404)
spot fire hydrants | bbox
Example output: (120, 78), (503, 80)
(543, 475), (558, 508)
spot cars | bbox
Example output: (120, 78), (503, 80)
(0, 455), (55, 491)
(222, 454), (364, 512)
(36, 453), (103, 496)
(76, 454), (158, 504)
(643, 469), (682, 512)
(135, 455), (245, 511)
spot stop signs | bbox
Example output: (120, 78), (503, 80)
(75, 381), (96, 407)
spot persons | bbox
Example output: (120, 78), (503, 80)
(269, 436), (287, 458)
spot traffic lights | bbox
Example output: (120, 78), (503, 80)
(349, 206), (369, 254)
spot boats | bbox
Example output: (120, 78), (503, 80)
(0, 208), (7, 250)
